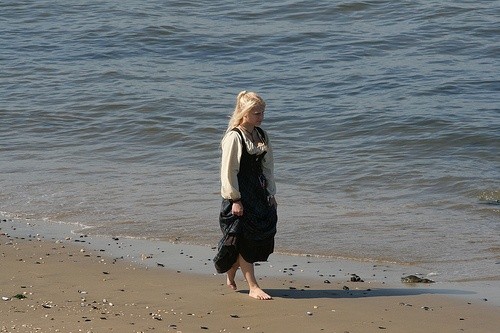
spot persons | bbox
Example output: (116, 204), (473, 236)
(214, 89), (279, 301)
